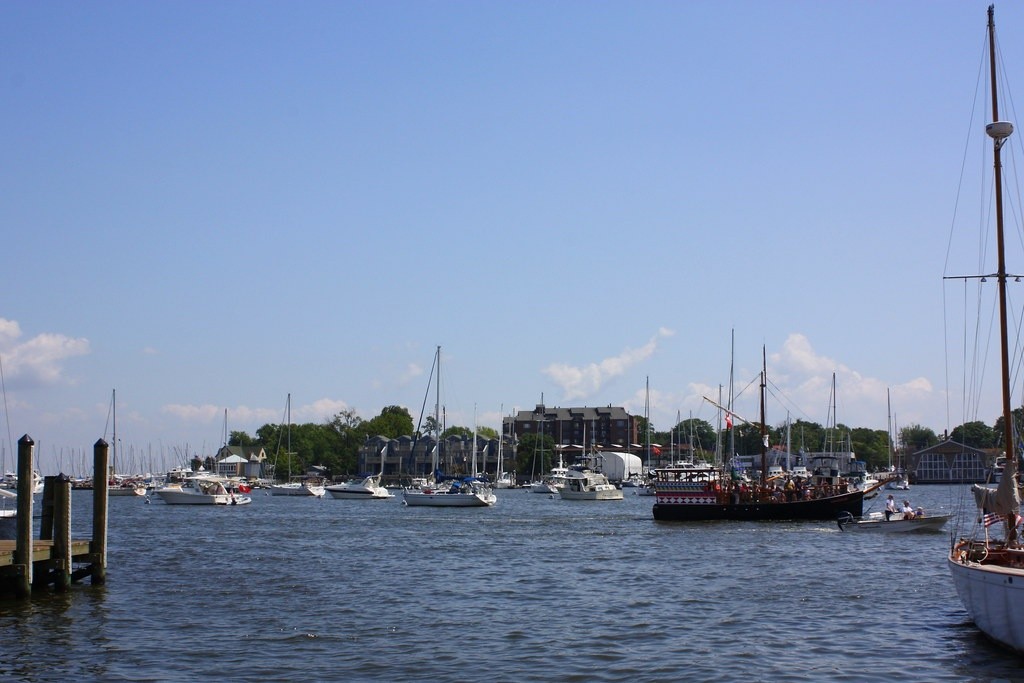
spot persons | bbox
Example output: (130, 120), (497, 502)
(726, 472), (859, 504)
(902, 500), (916, 520)
(885, 494), (895, 521)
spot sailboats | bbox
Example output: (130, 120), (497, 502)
(522, 323), (912, 524)
(942, 3), (1023, 663)
(0, 343), (518, 520)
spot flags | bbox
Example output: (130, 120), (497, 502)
(724, 415), (733, 430)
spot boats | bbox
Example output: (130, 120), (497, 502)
(837, 510), (959, 532)
